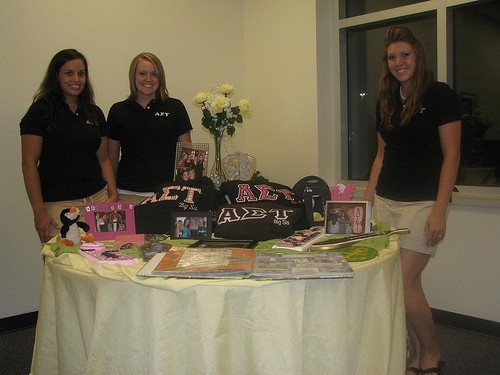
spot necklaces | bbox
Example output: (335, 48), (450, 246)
(398, 85), (417, 102)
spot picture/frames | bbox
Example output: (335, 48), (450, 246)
(170, 211), (212, 240)
(324, 200), (372, 235)
(83, 201), (136, 241)
(172, 142), (208, 182)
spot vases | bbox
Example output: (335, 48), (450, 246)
(210, 136), (230, 189)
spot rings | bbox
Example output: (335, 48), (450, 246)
(438, 233), (442, 237)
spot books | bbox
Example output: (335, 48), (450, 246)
(136, 228), (356, 280)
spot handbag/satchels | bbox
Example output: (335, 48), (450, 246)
(134, 177), (216, 234)
(292, 176), (331, 225)
(218, 179), (309, 229)
(214, 204), (302, 239)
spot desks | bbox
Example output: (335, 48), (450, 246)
(30, 220), (410, 375)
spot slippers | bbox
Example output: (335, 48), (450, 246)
(405, 359), (446, 375)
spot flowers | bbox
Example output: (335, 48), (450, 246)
(193, 83), (251, 177)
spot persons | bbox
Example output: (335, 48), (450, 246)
(327, 206), (363, 234)
(363, 26), (462, 375)
(95, 211), (126, 232)
(18, 49), (120, 264)
(180, 149), (204, 181)
(175, 216), (207, 238)
(106, 52), (193, 206)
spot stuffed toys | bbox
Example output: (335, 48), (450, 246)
(59, 205), (95, 246)
